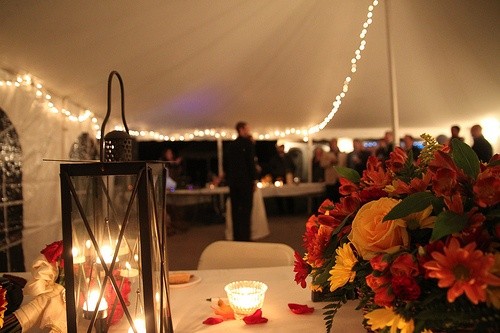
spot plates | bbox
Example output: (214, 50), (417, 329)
(168, 275), (200, 287)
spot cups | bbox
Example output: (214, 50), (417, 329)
(225, 280), (269, 319)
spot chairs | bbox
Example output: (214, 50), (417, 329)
(196, 240), (297, 271)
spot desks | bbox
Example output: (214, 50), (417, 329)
(0, 266), (367, 333)
(260, 181), (327, 214)
(165, 186), (229, 224)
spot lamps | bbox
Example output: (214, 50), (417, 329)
(59, 71), (174, 333)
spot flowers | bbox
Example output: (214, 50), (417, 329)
(294, 133), (500, 333)
(40, 240), (131, 326)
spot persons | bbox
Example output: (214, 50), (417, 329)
(449, 125), (464, 148)
(165, 148), (185, 186)
(330, 139), (340, 166)
(228, 121), (262, 242)
(312, 146), (325, 182)
(268, 144), (297, 213)
(346, 138), (371, 178)
(470, 125), (493, 164)
(405, 135), (421, 160)
(375, 132), (394, 174)
